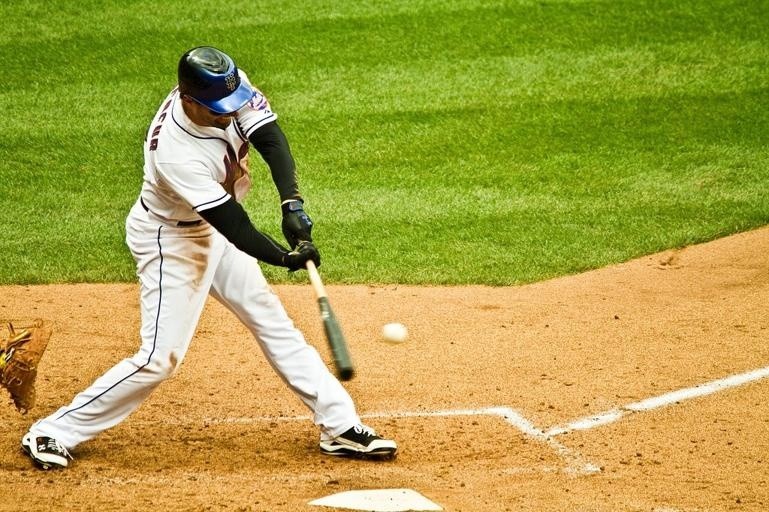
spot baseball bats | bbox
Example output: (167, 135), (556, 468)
(306, 259), (354, 381)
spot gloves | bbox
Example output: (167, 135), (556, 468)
(284, 241), (320, 272)
(141, 197), (201, 226)
(282, 201), (312, 250)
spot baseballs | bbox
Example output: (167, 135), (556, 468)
(384, 324), (406, 343)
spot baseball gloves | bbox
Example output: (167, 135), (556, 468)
(0, 319), (52, 415)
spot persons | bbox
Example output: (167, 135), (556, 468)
(21, 45), (397, 471)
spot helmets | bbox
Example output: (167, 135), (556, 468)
(178, 46), (256, 114)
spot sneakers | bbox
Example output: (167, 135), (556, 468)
(320, 424), (398, 456)
(20, 432), (74, 471)
